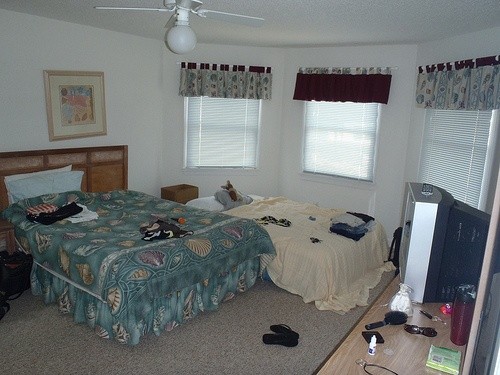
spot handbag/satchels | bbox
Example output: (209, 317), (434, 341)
(0, 250), (34, 300)
(0, 293), (11, 320)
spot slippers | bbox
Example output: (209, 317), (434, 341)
(262, 324), (300, 347)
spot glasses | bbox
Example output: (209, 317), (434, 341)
(404, 324), (437, 337)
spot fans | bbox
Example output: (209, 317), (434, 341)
(94, 0), (265, 28)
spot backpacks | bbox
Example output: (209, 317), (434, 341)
(384, 227), (402, 268)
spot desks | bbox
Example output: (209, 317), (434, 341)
(315, 276), (468, 375)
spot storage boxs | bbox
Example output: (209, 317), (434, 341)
(425, 344), (463, 375)
(161, 184), (199, 205)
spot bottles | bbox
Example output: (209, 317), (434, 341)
(368, 335), (377, 356)
(449, 285), (476, 346)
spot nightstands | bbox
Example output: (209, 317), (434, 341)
(0, 218), (16, 256)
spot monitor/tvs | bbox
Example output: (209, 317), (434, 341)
(398, 182), (500, 304)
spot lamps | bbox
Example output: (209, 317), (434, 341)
(166, 10), (197, 54)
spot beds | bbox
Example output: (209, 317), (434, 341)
(0, 145), (278, 349)
(186, 181), (396, 314)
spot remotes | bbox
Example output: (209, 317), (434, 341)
(421, 183), (433, 195)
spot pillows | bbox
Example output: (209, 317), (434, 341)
(185, 193), (252, 212)
(4, 165), (84, 206)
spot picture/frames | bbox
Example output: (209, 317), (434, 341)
(43, 69), (109, 141)
